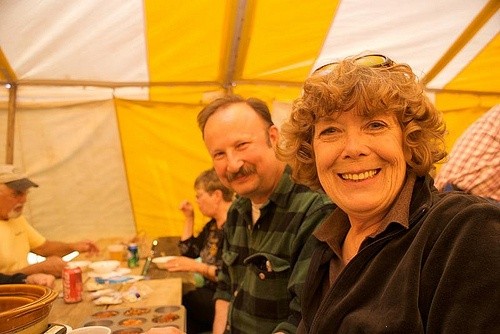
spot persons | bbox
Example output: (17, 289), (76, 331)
(274, 50), (500, 334)
(155, 168), (238, 334)
(197, 94), (334, 334)
(0, 164), (99, 277)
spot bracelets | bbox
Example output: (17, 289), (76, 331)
(204, 262), (209, 277)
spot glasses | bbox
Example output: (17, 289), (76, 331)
(311, 54), (397, 77)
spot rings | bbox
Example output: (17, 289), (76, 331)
(175, 263), (179, 266)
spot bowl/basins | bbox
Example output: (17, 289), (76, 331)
(66, 326), (112, 334)
(90, 259), (121, 272)
(151, 256), (175, 269)
(0, 284), (58, 334)
(64, 260), (90, 273)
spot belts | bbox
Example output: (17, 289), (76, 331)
(443, 184), (458, 191)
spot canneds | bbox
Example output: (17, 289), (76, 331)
(127, 243), (139, 268)
(62, 264), (83, 303)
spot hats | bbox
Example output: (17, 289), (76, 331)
(0, 163), (39, 189)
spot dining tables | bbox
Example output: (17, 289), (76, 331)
(48, 236), (182, 330)
